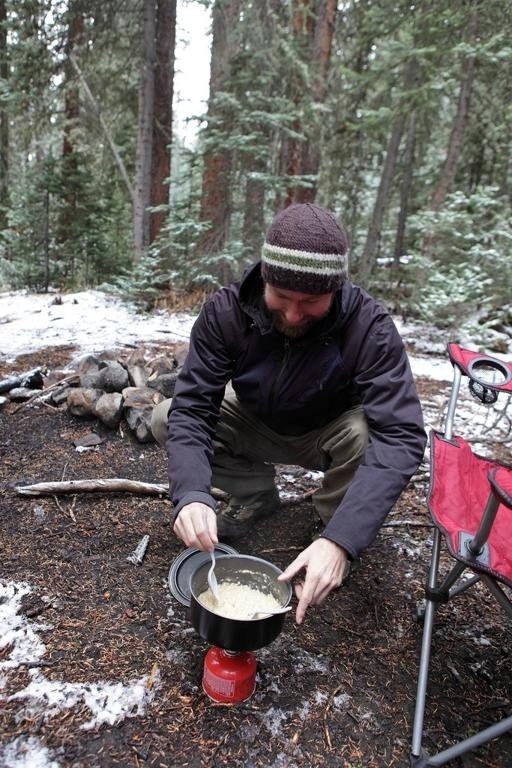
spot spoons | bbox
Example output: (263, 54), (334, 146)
(207, 549), (221, 601)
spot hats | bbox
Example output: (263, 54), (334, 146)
(261, 203), (348, 294)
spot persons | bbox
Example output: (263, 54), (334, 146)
(150, 203), (428, 625)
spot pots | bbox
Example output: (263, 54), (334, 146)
(189, 553), (293, 651)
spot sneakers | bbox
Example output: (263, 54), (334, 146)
(217, 487), (282, 541)
(308, 504), (352, 587)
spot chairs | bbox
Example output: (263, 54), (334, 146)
(409, 338), (512, 768)
